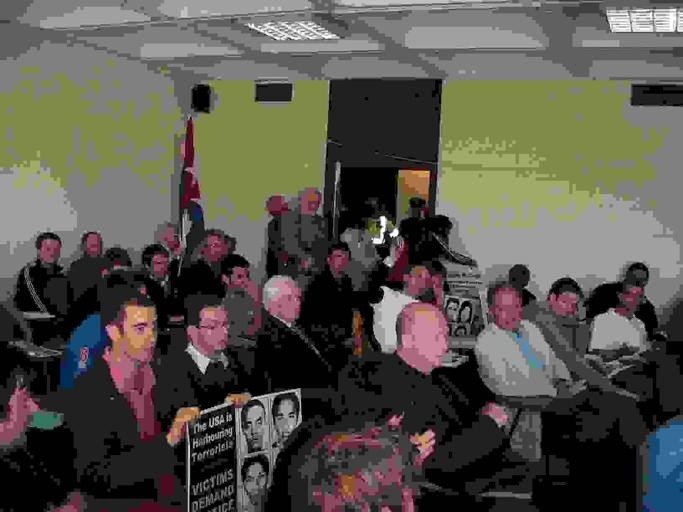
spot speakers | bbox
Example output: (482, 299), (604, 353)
(190, 83), (210, 113)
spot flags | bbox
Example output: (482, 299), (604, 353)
(178, 113), (205, 251)
